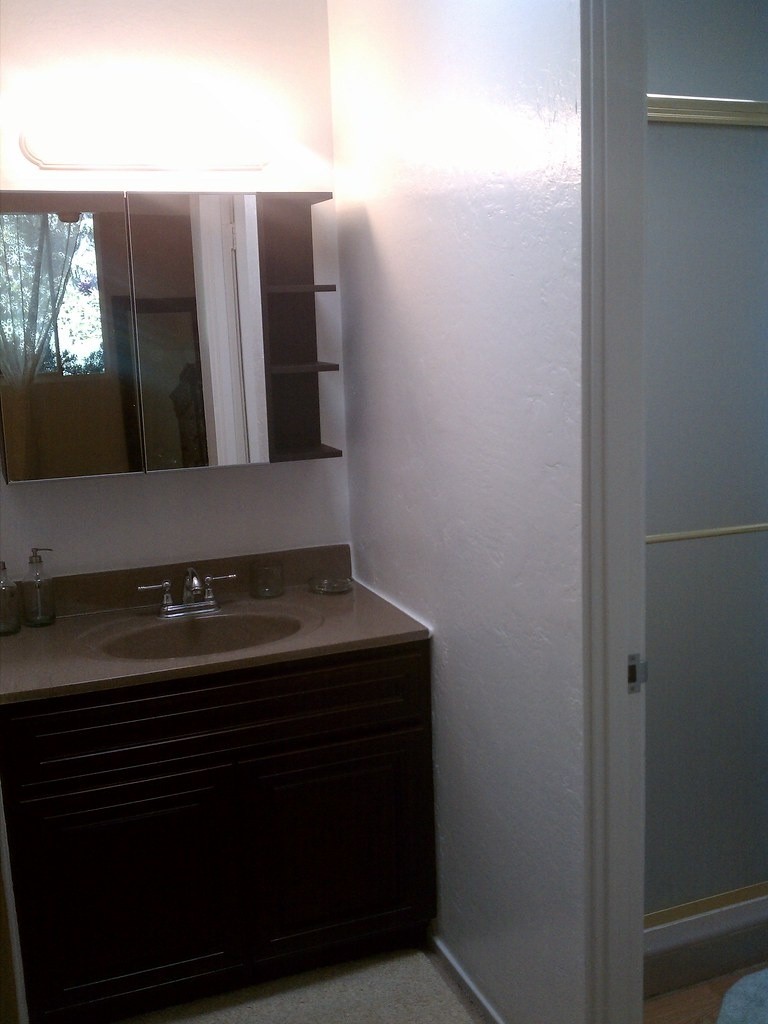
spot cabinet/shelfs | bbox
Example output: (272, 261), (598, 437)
(0, 638), (435, 1024)
(257, 191), (343, 464)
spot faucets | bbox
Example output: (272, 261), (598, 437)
(182, 565), (204, 605)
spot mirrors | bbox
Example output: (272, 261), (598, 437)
(0, 190), (209, 484)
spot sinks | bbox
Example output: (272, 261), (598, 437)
(73, 610), (301, 661)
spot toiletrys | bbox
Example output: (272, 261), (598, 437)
(0, 560), (21, 635)
(21, 545), (58, 627)
